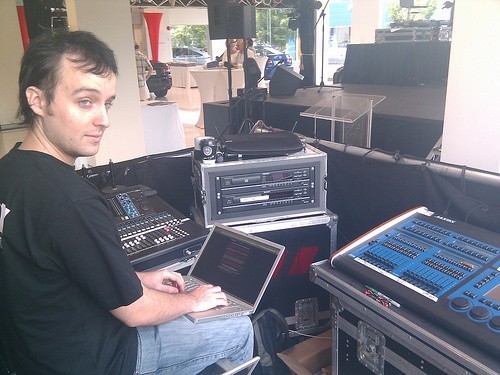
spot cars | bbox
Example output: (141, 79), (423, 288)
(172, 46), (211, 67)
(249, 42), (293, 81)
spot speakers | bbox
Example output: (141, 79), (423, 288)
(206, 2), (256, 40)
(270, 64), (305, 96)
(203, 100), (244, 138)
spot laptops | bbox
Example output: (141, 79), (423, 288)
(163, 223), (286, 324)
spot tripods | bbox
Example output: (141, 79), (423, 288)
(312, 0), (343, 88)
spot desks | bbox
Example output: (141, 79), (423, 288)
(171, 66), (203, 87)
(143, 101), (186, 155)
(190, 68), (245, 128)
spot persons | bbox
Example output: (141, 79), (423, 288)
(134, 40), (153, 100)
(0, 28), (254, 375)
(222, 37), (255, 66)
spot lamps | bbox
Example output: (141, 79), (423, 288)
(249, 0), (283, 9)
(288, 16), (298, 31)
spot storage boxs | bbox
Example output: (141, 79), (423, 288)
(186, 206), (339, 330)
(328, 291), (479, 375)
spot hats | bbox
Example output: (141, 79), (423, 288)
(134, 41), (139, 45)
